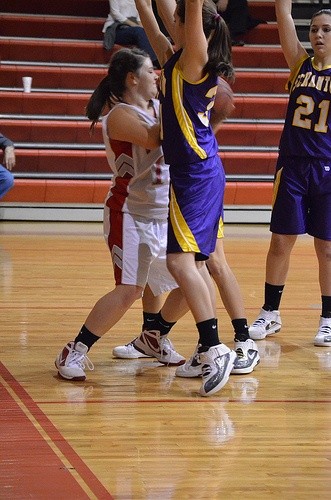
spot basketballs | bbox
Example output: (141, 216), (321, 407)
(209, 76), (232, 123)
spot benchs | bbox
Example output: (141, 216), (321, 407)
(0, 0), (330, 209)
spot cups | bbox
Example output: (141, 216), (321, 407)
(22, 77), (32, 93)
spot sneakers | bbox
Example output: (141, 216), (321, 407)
(232, 339), (260, 373)
(200, 344), (237, 395)
(133, 330), (186, 366)
(113, 338), (151, 358)
(53, 340), (94, 382)
(248, 308), (282, 340)
(314, 316), (330, 346)
(176, 345), (209, 378)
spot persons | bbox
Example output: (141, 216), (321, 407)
(54, 0), (262, 396)
(0, 132), (16, 198)
(249, 0), (331, 348)
